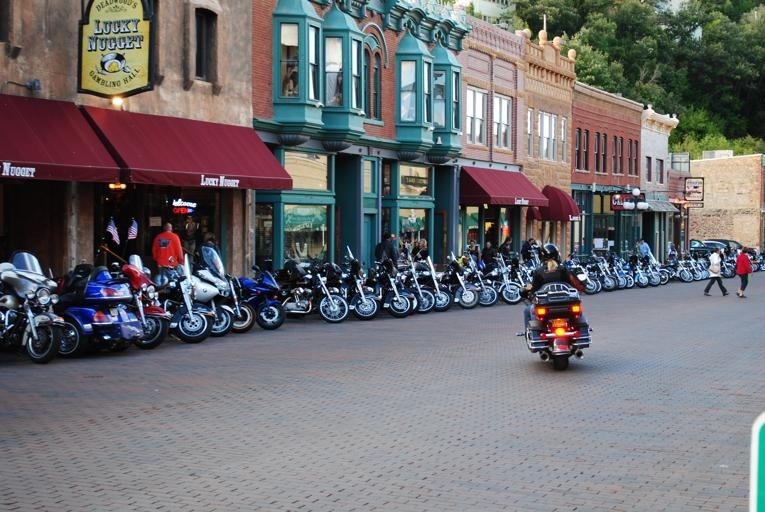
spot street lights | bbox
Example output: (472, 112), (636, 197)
(623, 188), (648, 256)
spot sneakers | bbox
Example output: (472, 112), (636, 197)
(702, 291), (749, 299)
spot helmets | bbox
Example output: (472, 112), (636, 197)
(541, 244), (559, 261)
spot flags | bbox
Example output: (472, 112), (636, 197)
(106, 220), (121, 245)
(128, 220), (138, 240)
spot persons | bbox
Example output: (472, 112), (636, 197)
(152, 222), (184, 293)
(375, 234), (397, 269)
(283, 63), (292, 93)
(639, 239), (652, 261)
(184, 215), (197, 261)
(523, 244), (585, 326)
(704, 246), (752, 297)
(333, 69), (342, 94)
(411, 237), (428, 262)
(468, 237), (534, 264)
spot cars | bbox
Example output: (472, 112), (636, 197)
(690, 238), (744, 257)
